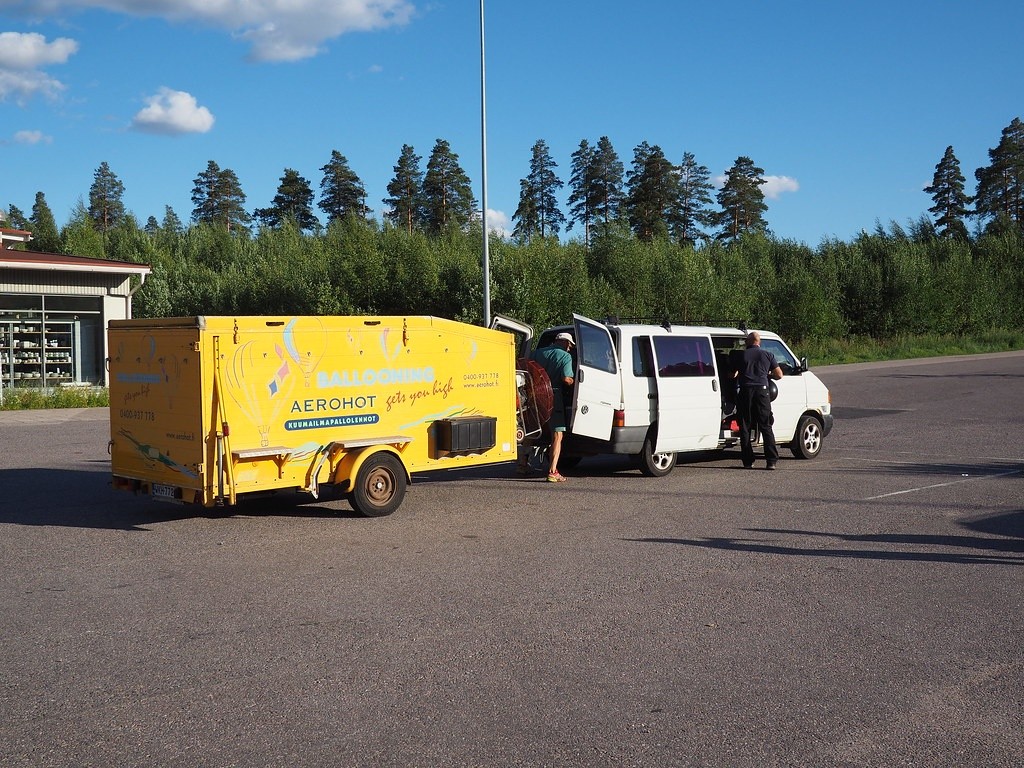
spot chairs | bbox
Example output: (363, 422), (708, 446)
(730, 349), (745, 366)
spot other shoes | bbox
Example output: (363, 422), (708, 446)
(744, 464), (754, 468)
(766, 463), (776, 469)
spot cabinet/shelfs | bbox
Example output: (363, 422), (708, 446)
(0, 318), (82, 390)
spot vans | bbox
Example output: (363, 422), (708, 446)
(486, 314), (836, 480)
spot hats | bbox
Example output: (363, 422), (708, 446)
(556, 333), (576, 345)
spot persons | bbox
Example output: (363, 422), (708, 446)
(529, 333), (575, 482)
(727, 332), (783, 471)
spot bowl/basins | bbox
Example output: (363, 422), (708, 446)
(15, 373), (21, 378)
(4, 374), (11, 379)
(20, 329), (28, 333)
(27, 327), (35, 332)
(33, 373), (40, 378)
(22, 374), (33, 378)
(64, 373), (70, 377)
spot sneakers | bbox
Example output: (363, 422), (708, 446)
(546, 470), (566, 482)
